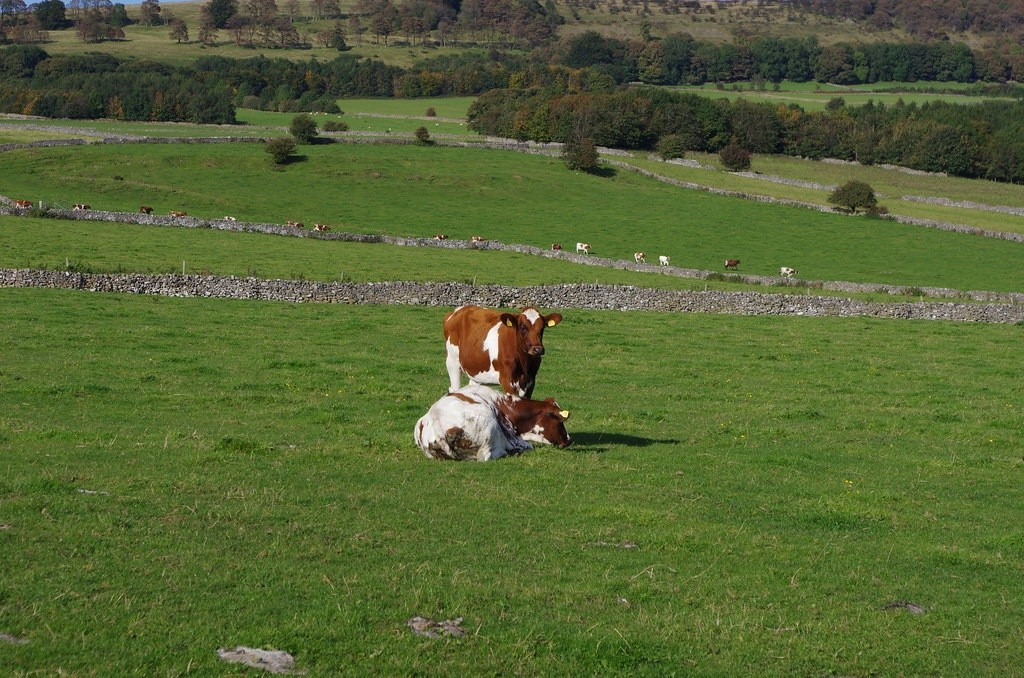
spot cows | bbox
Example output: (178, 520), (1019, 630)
(779, 266), (799, 280)
(169, 210), (188, 220)
(430, 233), (449, 241)
(72, 204), (91, 213)
(140, 206), (154, 215)
(634, 252), (647, 265)
(14, 199), (34, 210)
(659, 255), (671, 266)
(222, 215), (237, 223)
(470, 235), (484, 244)
(723, 258), (740, 271)
(312, 224), (331, 233)
(576, 242), (593, 256)
(550, 244), (564, 252)
(413, 305), (572, 463)
(284, 220), (305, 230)
